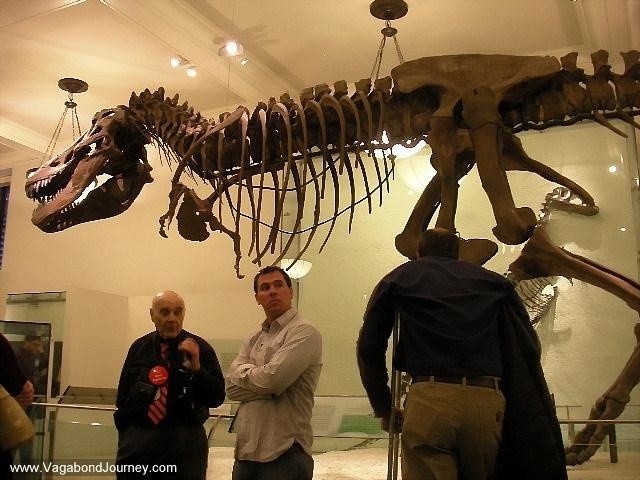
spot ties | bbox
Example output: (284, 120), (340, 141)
(148, 341), (172, 426)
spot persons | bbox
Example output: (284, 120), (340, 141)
(113, 291), (226, 479)
(7, 335), (44, 471)
(0, 331), (36, 480)
(223, 264), (326, 480)
(353, 225), (518, 480)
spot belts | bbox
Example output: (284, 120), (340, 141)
(412, 373), (506, 392)
